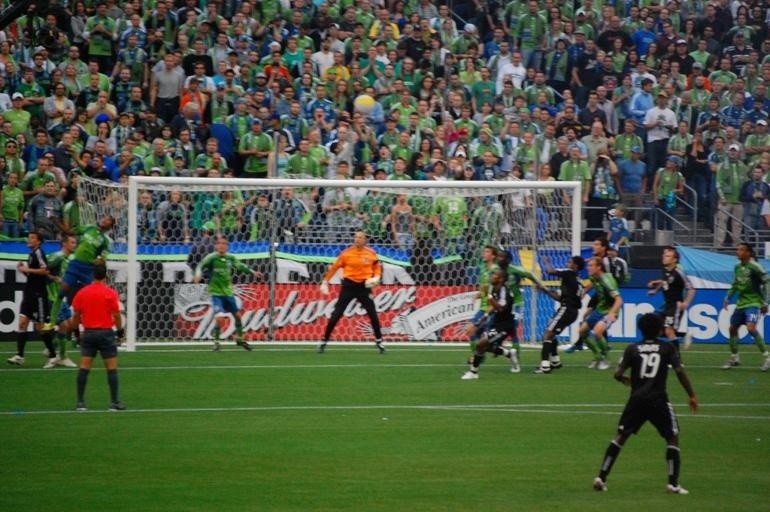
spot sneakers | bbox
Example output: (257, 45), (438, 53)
(460, 342), (684, 380)
(592, 478), (610, 493)
(213, 341), (220, 352)
(760, 356), (770, 371)
(237, 339), (254, 351)
(6, 347), (78, 369)
(317, 339), (327, 353)
(76, 401), (89, 411)
(722, 356), (741, 369)
(667, 484), (689, 495)
(375, 339), (385, 354)
(109, 403), (126, 412)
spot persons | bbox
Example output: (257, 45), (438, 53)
(310, 231), (390, 355)
(720, 241), (770, 373)
(40, 213), (116, 331)
(607, 202), (633, 269)
(592, 313), (699, 496)
(193, 236), (265, 354)
(70, 258), (127, 413)
(0, 2), (770, 250)
(7, 231), (60, 370)
(460, 236), (697, 381)
(41, 229), (77, 369)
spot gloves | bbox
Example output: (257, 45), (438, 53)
(319, 280), (331, 295)
(365, 276), (380, 288)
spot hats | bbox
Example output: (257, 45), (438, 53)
(0, 0), (770, 224)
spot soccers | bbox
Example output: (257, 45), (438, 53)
(354, 94), (376, 115)
